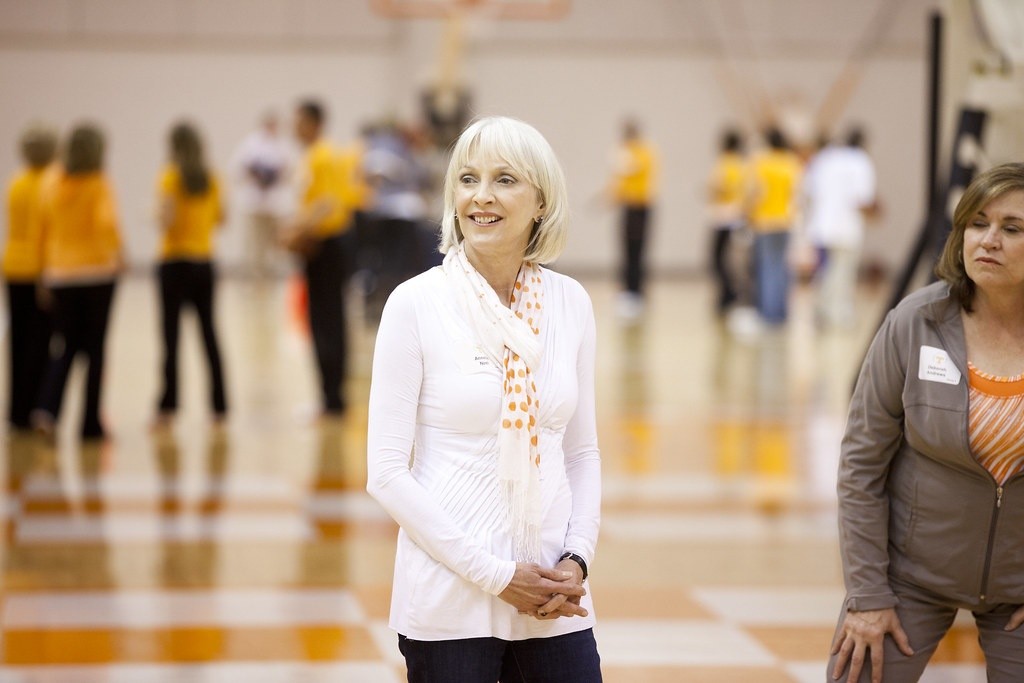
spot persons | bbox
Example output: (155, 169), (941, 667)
(237, 104), (428, 418)
(614, 120), (650, 312)
(707, 127), (883, 334)
(154, 124), (229, 418)
(365, 117), (602, 683)
(825, 161), (1024, 683)
(5, 122), (126, 444)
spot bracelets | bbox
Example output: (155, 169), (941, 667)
(558, 553), (588, 586)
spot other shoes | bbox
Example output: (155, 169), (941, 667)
(205, 395), (234, 428)
(156, 389), (181, 417)
(30, 406), (54, 443)
(317, 392), (352, 420)
(76, 414), (111, 445)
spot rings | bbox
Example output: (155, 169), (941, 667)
(541, 612), (548, 617)
